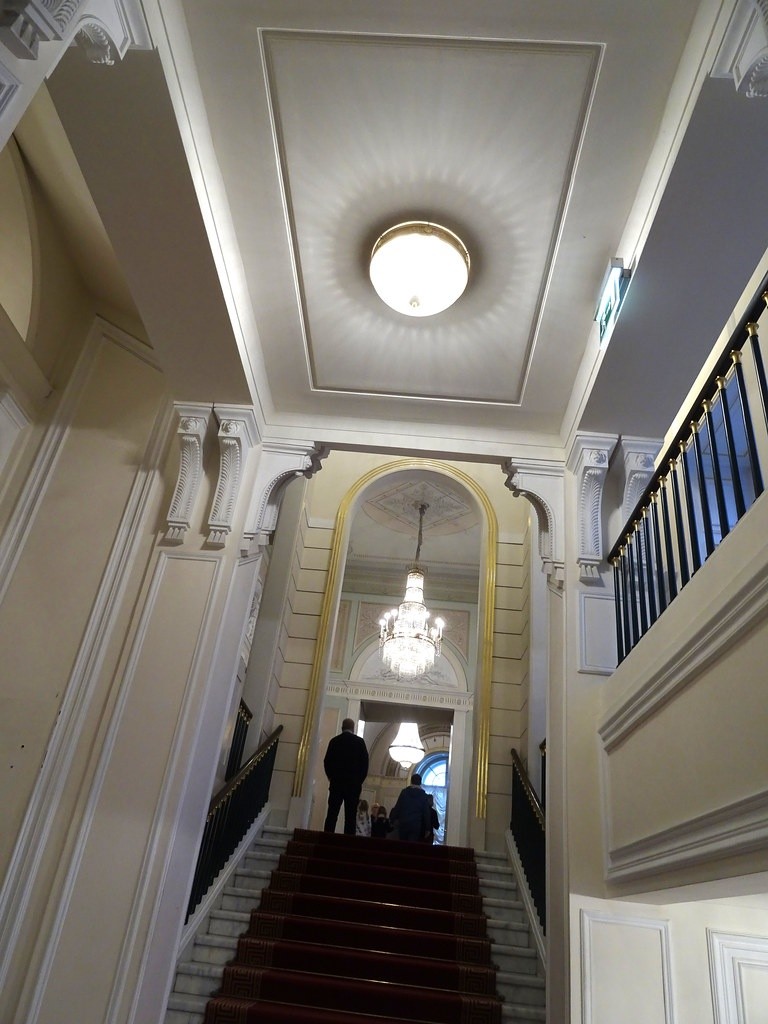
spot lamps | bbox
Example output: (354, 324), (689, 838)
(368, 218), (472, 318)
(378, 502), (447, 676)
(388, 722), (427, 771)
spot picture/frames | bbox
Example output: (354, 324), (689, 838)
(356, 788), (376, 836)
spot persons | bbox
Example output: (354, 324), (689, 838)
(324, 718), (369, 835)
(356, 774), (440, 844)
